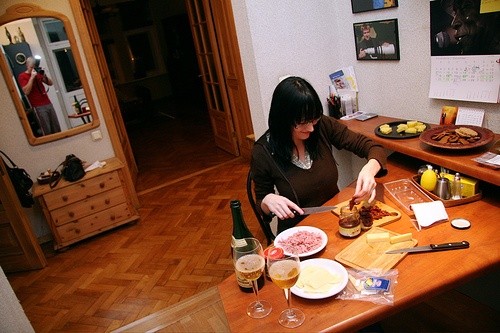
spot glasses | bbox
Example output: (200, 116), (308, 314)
(296, 114), (321, 128)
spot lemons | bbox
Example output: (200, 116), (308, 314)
(420, 169), (437, 192)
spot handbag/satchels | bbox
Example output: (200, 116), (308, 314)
(9, 166), (33, 192)
(49, 153), (87, 188)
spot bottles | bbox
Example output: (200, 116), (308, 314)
(18, 27), (24, 41)
(73, 95), (81, 115)
(229, 200), (264, 294)
(339, 205), (362, 239)
(452, 172), (462, 200)
(5, 27), (12, 44)
(435, 177), (451, 199)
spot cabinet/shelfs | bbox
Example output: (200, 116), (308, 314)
(31, 157), (142, 253)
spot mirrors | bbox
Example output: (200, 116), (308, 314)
(0, 3), (101, 146)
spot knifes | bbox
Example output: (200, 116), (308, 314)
(384, 241), (469, 255)
(270, 206), (339, 217)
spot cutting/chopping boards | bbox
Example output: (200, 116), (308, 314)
(334, 227), (419, 278)
(330, 196), (402, 232)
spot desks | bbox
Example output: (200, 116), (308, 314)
(219, 110), (500, 333)
(68, 110), (91, 124)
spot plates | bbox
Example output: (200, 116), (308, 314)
(289, 258), (348, 299)
(273, 226), (327, 258)
(374, 120), (431, 140)
(419, 125), (495, 151)
(37, 171), (60, 185)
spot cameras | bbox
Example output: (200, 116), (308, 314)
(33, 59), (45, 74)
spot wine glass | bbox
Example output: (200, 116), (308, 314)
(232, 237), (272, 320)
(267, 244), (305, 329)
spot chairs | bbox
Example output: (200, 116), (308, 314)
(246, 168), (279, 247)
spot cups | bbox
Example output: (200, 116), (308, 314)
(82, 107), (86, 113)
(14, 35), (19, 43)
(328, 103), (342, 119)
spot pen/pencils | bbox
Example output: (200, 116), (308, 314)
(325, 94), (335, 105)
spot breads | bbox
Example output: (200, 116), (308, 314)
(455, 127), (478, 137)
(397, 120), (426, 133)
(379, 123), (392, 134)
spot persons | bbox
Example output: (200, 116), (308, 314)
(451, 0), (500, 56)
(18, 57), (62, 136)
(357, 25), (390, 60)
(250, 77), (389, 240)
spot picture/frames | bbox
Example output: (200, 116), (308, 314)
(351, 0), (400, 60)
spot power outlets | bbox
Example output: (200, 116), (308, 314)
(90, 130), (103, 142)
